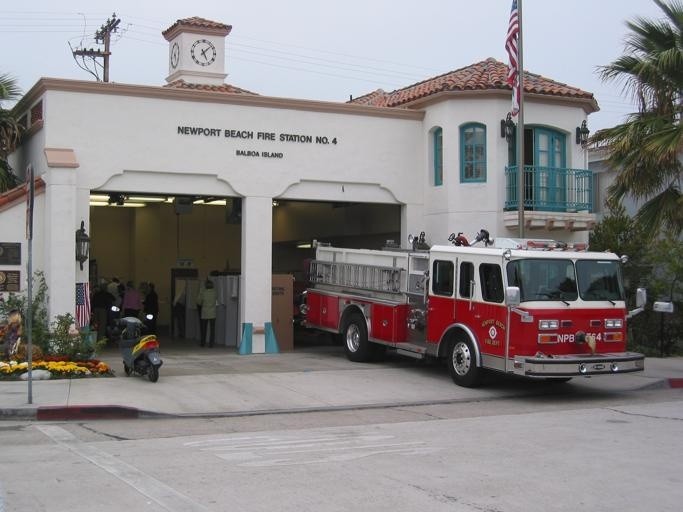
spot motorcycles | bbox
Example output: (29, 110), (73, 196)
(106, 305), (163, 383)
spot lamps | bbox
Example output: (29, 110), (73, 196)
(501, 112), (515, 143)
(576, 120), (590, 148)
(75, 221), (91, 270)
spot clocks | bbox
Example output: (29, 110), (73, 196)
(171, 42), (179, 69)
(191, 39), (216, 65)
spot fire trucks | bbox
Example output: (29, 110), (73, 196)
(296, 236), (644, 388)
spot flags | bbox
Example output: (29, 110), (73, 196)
(76, 282), (92, 327)
(504, 1), (519, 117)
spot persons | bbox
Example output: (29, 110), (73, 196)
(175, 289), (185, 335)
(122, 281), (141, 317)
(196, 280), (218, 348)
(107, 275), (124, 316)
(92, 290), (116, 338)
(140, 282), (160, 334)
